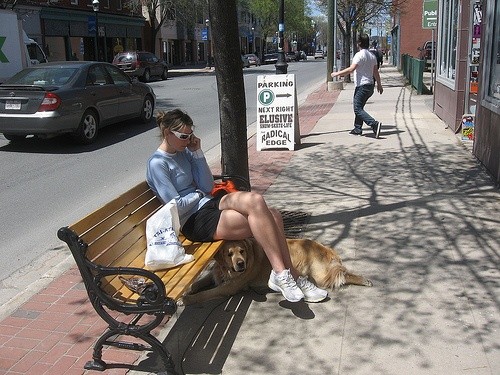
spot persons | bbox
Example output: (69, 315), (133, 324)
(146, 108), (328, 302)
(330, 34), (383, 139)
(369, 40), (383, 69)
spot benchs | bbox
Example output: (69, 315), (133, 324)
(57, 175), (250, 375)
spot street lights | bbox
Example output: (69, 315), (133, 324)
(206, 18), (211, 70)
(92, 0), (100, 62)
(252, 26), (254, 54)
(275, 32), (279, 51)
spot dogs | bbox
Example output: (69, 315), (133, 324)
(182, 236), (372, 306)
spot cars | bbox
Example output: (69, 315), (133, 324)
(314, 50), (324, 60)
(0, 60), (156, 143)
(241, 51), (306, 68)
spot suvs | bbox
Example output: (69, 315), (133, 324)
(112, 51), (170, 83)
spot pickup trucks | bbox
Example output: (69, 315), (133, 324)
(417, 41), (437, 72)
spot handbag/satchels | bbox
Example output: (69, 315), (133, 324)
(144, 199), (194, 270)
(211, 175), (239, 196)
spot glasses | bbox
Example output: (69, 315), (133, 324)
(170, 131), (194, 140)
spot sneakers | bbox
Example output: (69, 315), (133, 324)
(268, 267), (305, 303)
(297, 275), (328, 302)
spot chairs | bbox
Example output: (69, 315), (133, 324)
(87, 74), (101, 86)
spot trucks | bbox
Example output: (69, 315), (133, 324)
(0, 8), (48, 86)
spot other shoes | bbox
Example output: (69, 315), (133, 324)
(372, 121), (381, 139)
(348, 129), (361, 136)
